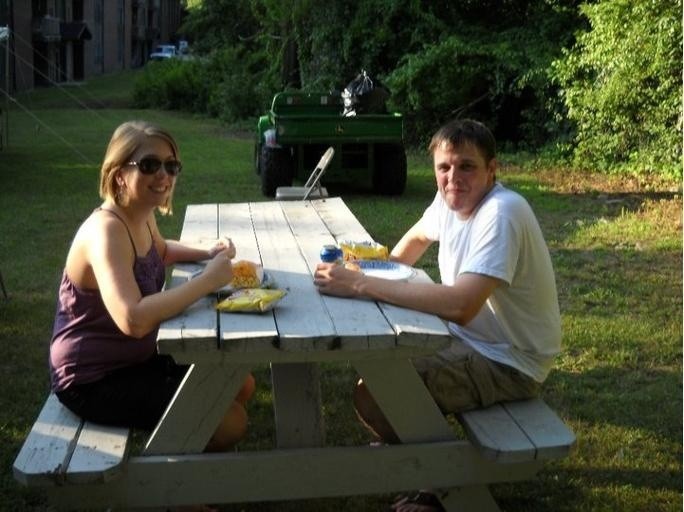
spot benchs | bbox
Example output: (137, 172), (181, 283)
(11, 281), (167, 492)
(459, 400), (580, 464)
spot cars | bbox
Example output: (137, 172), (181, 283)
(149, 43), (176, 60)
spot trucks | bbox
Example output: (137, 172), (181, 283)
(255, 85), (409, 195)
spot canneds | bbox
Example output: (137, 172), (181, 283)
(320, 244), (344, 267)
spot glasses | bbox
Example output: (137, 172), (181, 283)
(125, 157), (182, 176)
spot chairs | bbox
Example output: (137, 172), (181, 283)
(276, 147), (335, 200)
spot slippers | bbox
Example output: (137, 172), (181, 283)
(390, 488), (444, 511)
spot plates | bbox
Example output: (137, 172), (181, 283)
(345, 260), (416, 284)
(188, 268), (273, 296)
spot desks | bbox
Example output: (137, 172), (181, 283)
(14, 196), (507, 512)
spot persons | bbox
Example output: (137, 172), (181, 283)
(48, 120), (257, 510)
(313, 116), (561, 512)
